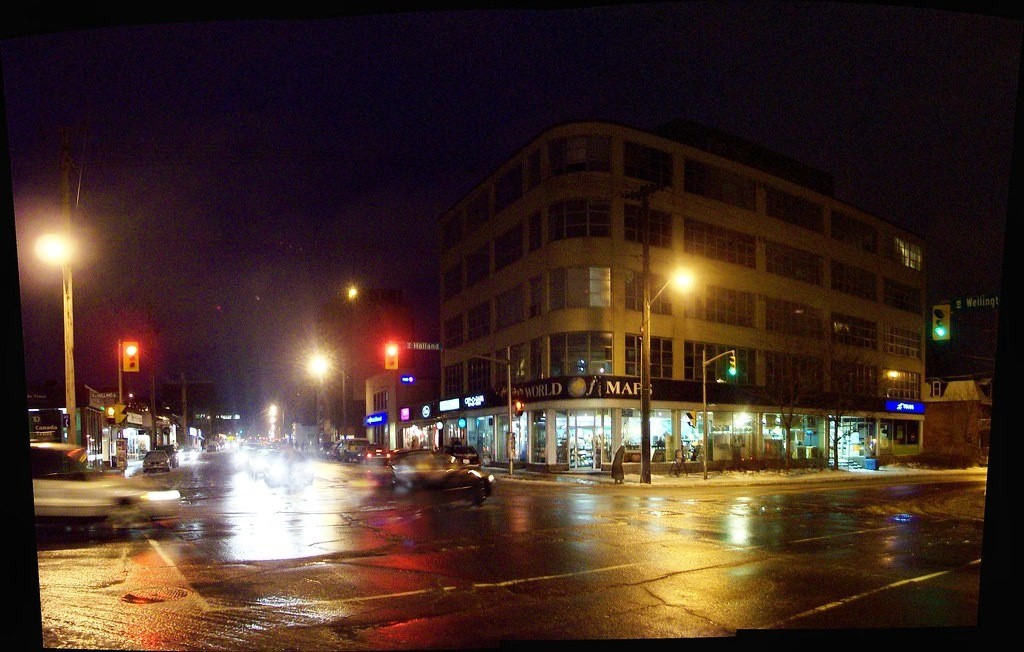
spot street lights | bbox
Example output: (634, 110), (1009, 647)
(638, 267), (694, 485)
(34, 229), (77, 446)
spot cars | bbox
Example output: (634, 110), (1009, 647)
(27, 432), (499, 531)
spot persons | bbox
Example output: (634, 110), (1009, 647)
(449, 437), (462, 447)
(611, 446), (626, 483)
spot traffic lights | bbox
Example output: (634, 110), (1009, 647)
(384, 342), (399, 370)
(930, 304), (951, 341)
(105, 406), (115, 418)
(120, 341), (140, 373)
(514, 400), (525, 417)
(728, 355), (738, 376)
(114, 403), (128, 424)
(685, 410), (696, 426)
